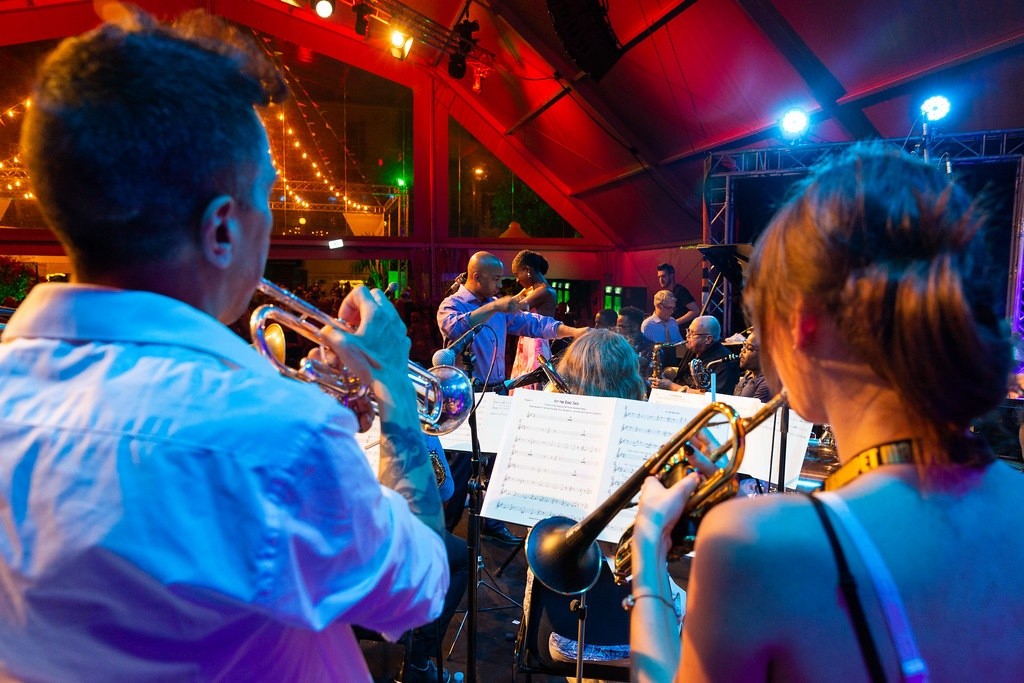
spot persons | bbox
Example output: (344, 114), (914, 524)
(227, 247), (1023, 401)
(627, 135), (1023, 683)
(434, 250), (590, 547)
(0, 22), (452, 683)
(544, 328), (648, 683)
(349, 403), (473, 683)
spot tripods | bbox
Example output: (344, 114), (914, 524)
(438, 388), (523, 663)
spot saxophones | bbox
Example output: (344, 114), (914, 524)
(650, 338), (687, 391)
(690, 352), (741, 391)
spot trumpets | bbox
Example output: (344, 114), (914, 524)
(526, 387), (790, 597)
(248, 279), (476, 433)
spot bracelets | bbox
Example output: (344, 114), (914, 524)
(622, 590), (678, 613)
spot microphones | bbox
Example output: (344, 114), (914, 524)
(946, 154), (953, 183)
(446, 272), (468, 297)
(433, 323), (486, 367)
(922, 113), (934, 164)
(384, 283), (398, 298)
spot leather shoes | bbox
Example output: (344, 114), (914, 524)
(393, 659), (451, 683)
(480, 527), (524, 545)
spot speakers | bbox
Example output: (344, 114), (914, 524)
(546, 0), (621, 75)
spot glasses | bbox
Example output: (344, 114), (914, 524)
(685, 328), (712, 338)
(659, 302), (675, 311)
(742, 340), (759, 353)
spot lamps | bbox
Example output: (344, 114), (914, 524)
(917, 94), (954, 123)
(311, 0), (336, 19)
(448, 41), (472, 78)
(389, 26), (413, 60)
(453, 18), (480, 52)
(353, 4), (374, 36)
(779, 106), (810, 140)
